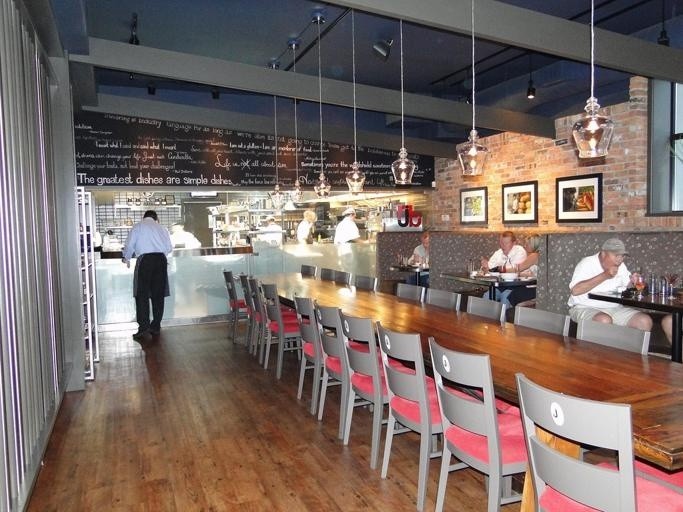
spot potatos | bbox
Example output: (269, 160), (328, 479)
(517, 195), (531, 214)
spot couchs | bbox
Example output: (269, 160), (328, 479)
(375, 232), (433, 291)
(428, 232), (536, 309)
(536, 230), (683, 336)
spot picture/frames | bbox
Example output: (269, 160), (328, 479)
(501, 179), (538, 224)
(555, 172), (601, 222)
(460, 187), (488, 225)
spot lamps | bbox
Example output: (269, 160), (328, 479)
(658, 1), (669, 46)
(464, 67), (472, 104)
(268, 38), (302, 206)
(312, 8), (330, 196)
(344, 9), (365, 195)
(525, 53), (535, 99)
(455, 1), (486, 177)
(390, 19), (414, 185)
(572, 0), (613, 159)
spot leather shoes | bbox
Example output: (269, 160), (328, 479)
(132, 327), (150, 338)
(149, 326), (161, 335)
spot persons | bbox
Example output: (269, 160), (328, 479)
(264, 215), (282, 245)
(507, 193), (520, 214)
(660, 314), (673, 345)
(464, 197), (475, 215)
(296, 209), (317, 245)
(480, 230), (528, 309)
(496, 230), (542, 325)
(122, 210), (174, 339)
(563, 188), (576, 212)
(169, 220), (202, 249)
(568, 237), (654, 332)
(333, 206), (361, 298)
(403, 231), (431, 289)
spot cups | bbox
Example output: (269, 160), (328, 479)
(513, 264), (519, 277)
(394, 248), (428, 272)
(647, 273), (676, 304)
(499, 265), (505, 273)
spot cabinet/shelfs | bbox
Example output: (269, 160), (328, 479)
(208, 201), (329, 246)
(104, 203), (184, 230)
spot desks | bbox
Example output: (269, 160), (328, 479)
(440, 273), (537, 300)
(588, 290), (681, 359)
(390, 266), (433, 282)
(257, 273), (683, 470)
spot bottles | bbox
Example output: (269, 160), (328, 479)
(467, 258), (474, 275)
(79, 221), (89, 372)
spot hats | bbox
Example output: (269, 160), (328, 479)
(303, 210), (317, 223)
(341, 208), (356, 216)
(602, 238), (629, 257)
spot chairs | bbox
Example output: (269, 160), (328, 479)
(396, 283), (425, 302)
(427, 337), (528, 512)
(515, 306), (570, 336)
(223, 270), (295, 367)
(313, 299), (400, 443)
(338, 309), (414, 470)
(576, 319), (651, 356)
(300, 266), (377, 290)
(292, 292), (355, 417)
(426, 288), (461, 311)
(466, 296), (506, 322)
(375, 321), (484, 512)
(513, 371), (681, 510)
(261, 284), (308, 379)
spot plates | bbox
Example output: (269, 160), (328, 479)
(468, 270), (534, 281)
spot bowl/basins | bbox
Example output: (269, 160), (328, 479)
(499, 273), (517, 282)
(292, 203), (309, 208)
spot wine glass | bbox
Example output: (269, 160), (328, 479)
(634, 276), (646, 299)
